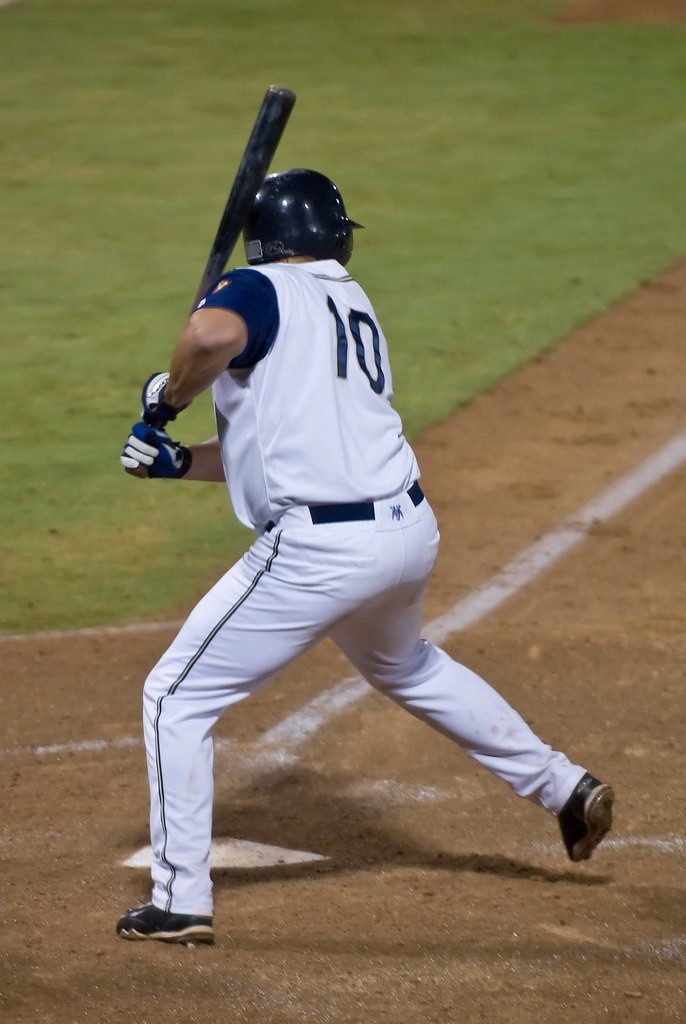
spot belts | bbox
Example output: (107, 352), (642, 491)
(308, 480), (423, 528)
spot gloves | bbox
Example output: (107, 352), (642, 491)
(141, 371), (184, 426)
(122, 422), (192, 480)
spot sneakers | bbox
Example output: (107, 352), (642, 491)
(116, 901), (215, 942)
(558, 771), (614, 861)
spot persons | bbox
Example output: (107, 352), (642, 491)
(115, 167), (613, 945)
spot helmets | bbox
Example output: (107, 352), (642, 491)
(244, 169), (364, 261)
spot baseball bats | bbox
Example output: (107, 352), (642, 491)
(124, 83), (298, 479)
